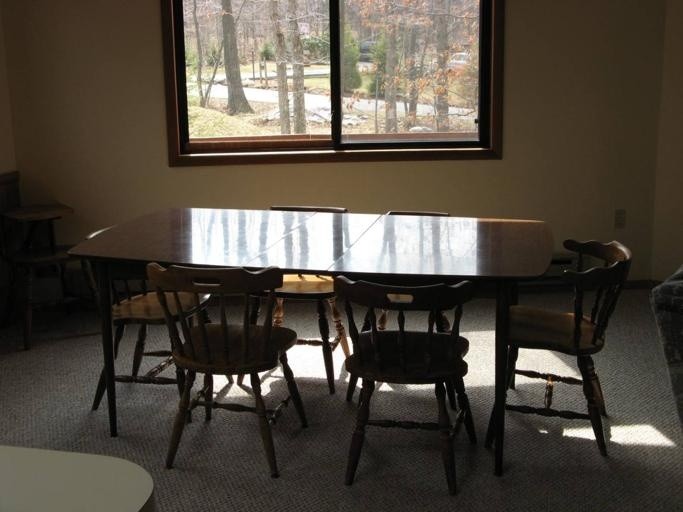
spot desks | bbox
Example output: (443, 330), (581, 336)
(0, 446), (156, 512)
